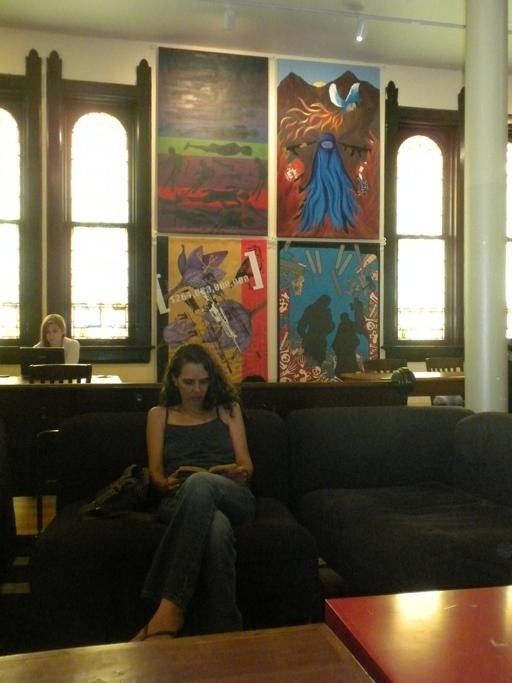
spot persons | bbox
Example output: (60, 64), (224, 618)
(31, 312), (80, 363)
(129, 344), (256, 644)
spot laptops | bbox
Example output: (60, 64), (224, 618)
(19, 347), (65, 378)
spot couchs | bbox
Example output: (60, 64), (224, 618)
(279, 405), (512, 592)
(34, 404), (323, 635)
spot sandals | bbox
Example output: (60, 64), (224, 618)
(128, 626), (178, 641)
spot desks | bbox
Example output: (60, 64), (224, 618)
(325, 585), (511, 683)
(335, 370), (467, 406)
(0, 621), (375, 682)
(0, 362), (155, 390)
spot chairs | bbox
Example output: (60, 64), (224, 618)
(17, 347), (94, 384)
(361, 356), (466, 406)
